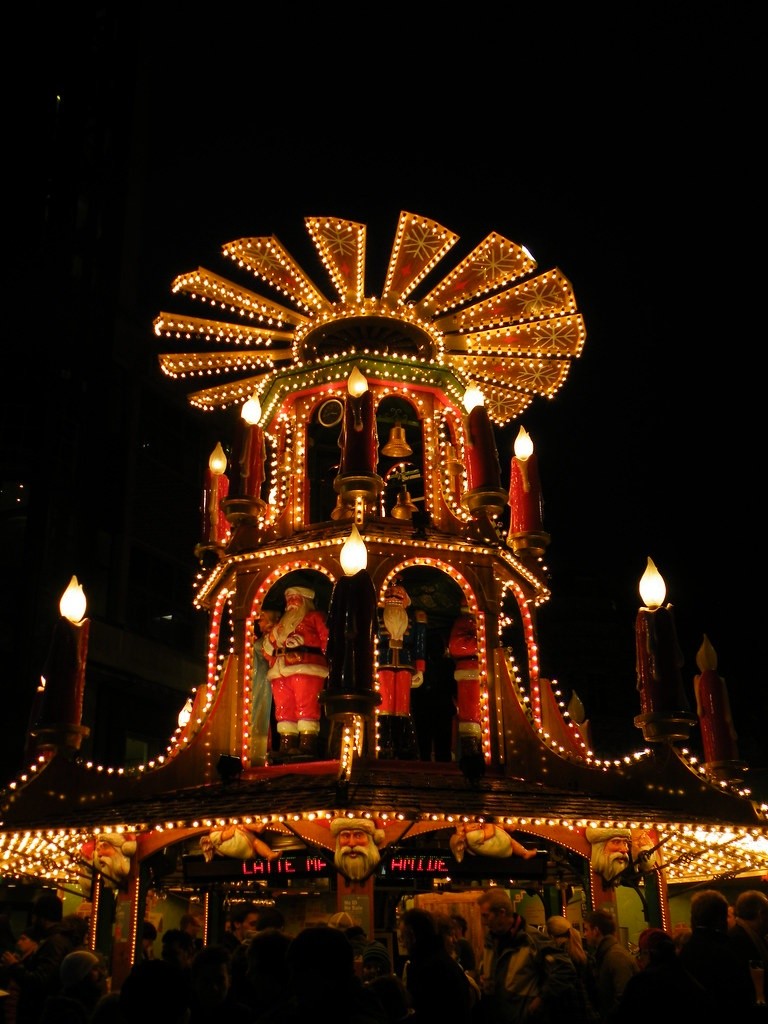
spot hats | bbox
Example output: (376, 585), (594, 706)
(361, 941), (391, 970)
(631, 928), (676, 956)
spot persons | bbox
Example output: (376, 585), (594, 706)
(444, 597), (486, 773)
(375, 578), (427, 760)
(248, 609), (280, 759)
(96, 833), (137, 887)
(262, 586), (330, 759)
(582, 825), (631, 886)
(329, 818), (386, 882)
(361, 889), (768, 1024)
(0, 891), (375, 1024)
